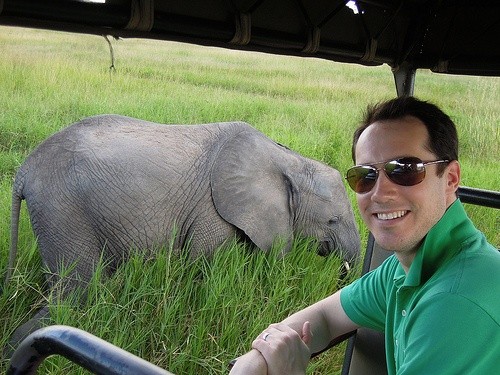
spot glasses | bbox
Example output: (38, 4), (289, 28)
(344, 156), (450, 194)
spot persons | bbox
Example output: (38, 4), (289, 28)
(226, 92), (500, 375)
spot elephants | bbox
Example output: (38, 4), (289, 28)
(6, 113), (363, 363)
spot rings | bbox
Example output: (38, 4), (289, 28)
(263, 332), (270, 340)
